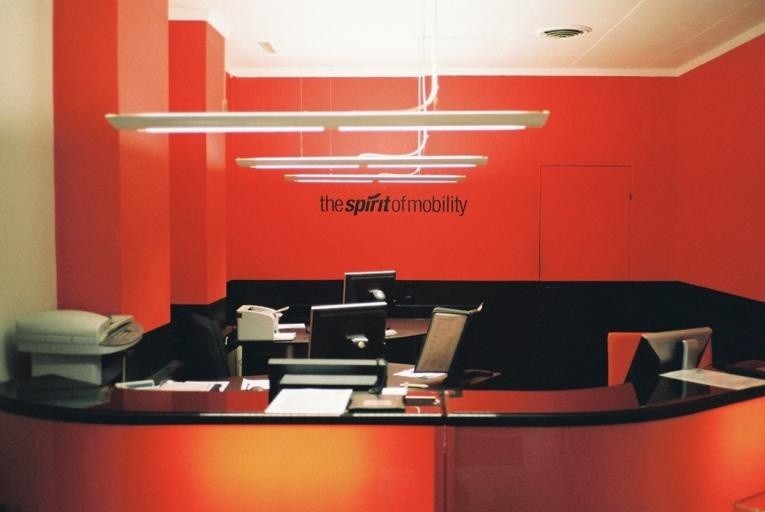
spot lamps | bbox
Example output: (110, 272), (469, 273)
(103, 0), (551, 134)
(281, 0), (468, 185)
(233, 0), (490, 169)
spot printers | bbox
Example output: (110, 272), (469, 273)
(236, 304), (297, 341)
(16, 309), (143, 385)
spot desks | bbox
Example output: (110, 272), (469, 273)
(386, 362), (503, 391)
(226, 308), (433, 380)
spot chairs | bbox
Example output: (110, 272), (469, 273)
(173, 311), (232, 379)
(607, 326), (714, 384)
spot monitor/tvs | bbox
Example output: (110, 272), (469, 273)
(308, 302), (387, 360)
(623, 326), (715, 384)
(342, 270), (395, 317)
(268, 359), (386, 392)
